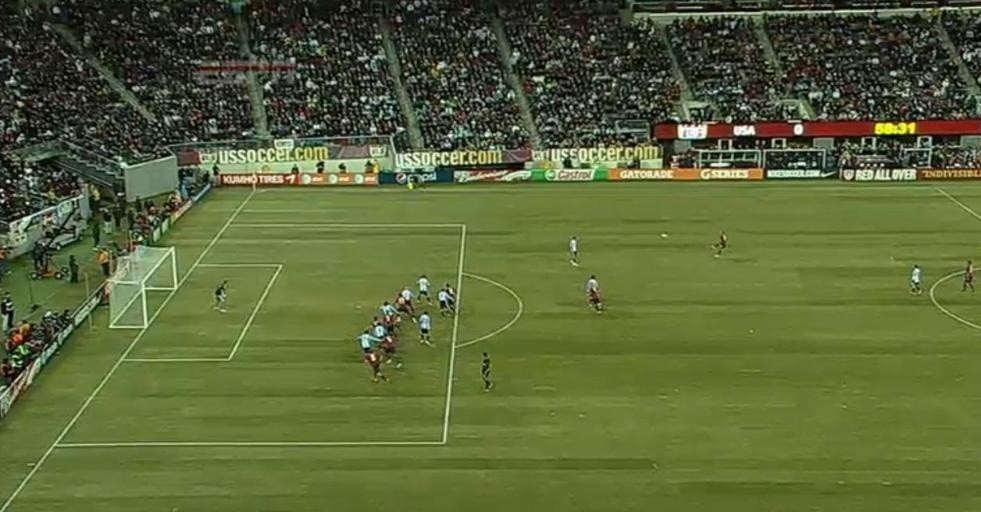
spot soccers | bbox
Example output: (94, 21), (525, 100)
(659, 234), (668, 239)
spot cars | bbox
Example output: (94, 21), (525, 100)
(36, 224), (84, 251)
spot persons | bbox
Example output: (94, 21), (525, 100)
(213, 279), (231, 313)
(570, 236), (580, 267)
(589, 287), (606, 315)
(911, 264), (922, 296)
(710, 228), (728, 260)
(356, 273), (457, 382)
(962, 260), (976, 293)
(586, 274), (600, 307)
(481, 351), (493, 393)
